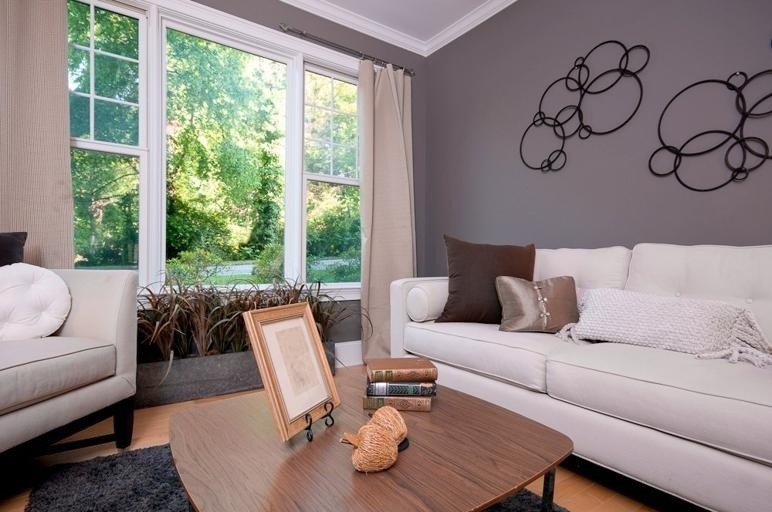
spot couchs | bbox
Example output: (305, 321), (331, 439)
(390, 243), (772, 511)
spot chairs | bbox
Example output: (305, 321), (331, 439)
(0, 231), (138, 499)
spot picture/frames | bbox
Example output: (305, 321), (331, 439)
(241, 301), (341, 444)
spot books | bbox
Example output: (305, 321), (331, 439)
(363, 377), (438, 396)
(362, 392), (432, 411)
(363, 355), (438, 383)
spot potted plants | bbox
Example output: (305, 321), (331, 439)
(137, 269), (373, 409)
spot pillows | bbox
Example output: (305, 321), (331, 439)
(496, 274), (579, 333)
(533, 245), (635, 287)
(556, 288), (772, 366)
(0, 262), (72, 342)
(437, 232), (536, 324)
(624, 243), (772, 341)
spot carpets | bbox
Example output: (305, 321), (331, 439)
(23, 440), (569, 511)
